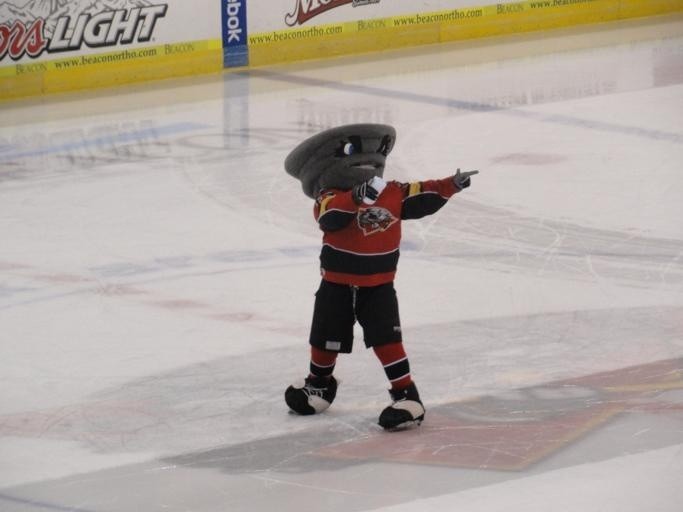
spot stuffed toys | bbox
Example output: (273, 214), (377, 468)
(284, 123), (479, 433)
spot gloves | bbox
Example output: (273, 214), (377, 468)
(359, 182), (378, 202)
(454, 168), (478, 189)
